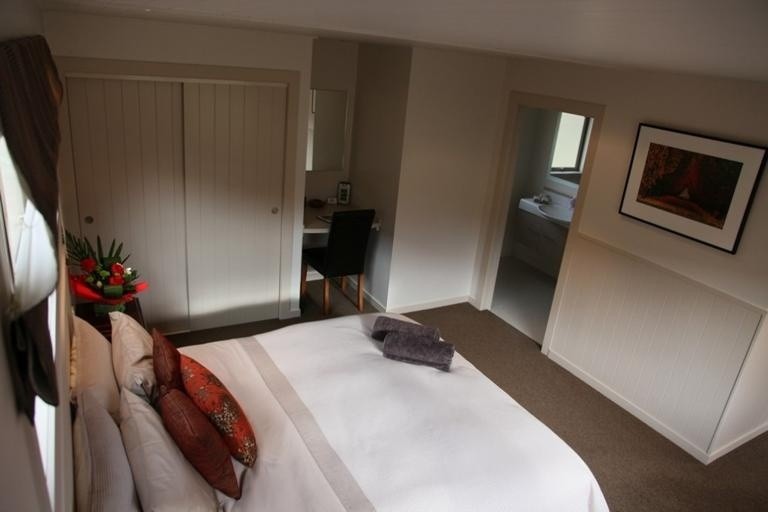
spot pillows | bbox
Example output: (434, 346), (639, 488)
(179, 353), (258, 467)
(106, 311), (160, 409)
(158, 384), (242, 500)
(120, 384), (218, 511)
(69, 313), (121, 426)
(152, 327), (185, 392)
(72, 391), (141, 511)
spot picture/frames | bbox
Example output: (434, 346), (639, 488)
(618, 123), (768, 255)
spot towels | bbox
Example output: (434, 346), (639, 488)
(371, 316), (440, 343)
(383, 332), (455, 372)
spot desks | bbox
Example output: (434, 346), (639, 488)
(304, 202), (380, 282)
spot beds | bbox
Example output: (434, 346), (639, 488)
(54, 208), (613, 512)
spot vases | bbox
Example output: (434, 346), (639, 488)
(93, 304), (126, 318)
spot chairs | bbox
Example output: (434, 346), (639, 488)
(301, 209), (376, 317)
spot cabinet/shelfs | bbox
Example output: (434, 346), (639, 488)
(51, 57), (300, 338)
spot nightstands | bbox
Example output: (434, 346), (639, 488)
(75, 297), (145, 343)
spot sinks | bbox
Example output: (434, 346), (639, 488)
(538, 202), (573, 222)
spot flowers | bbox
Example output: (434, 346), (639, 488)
(65, 229), (148, 305)
